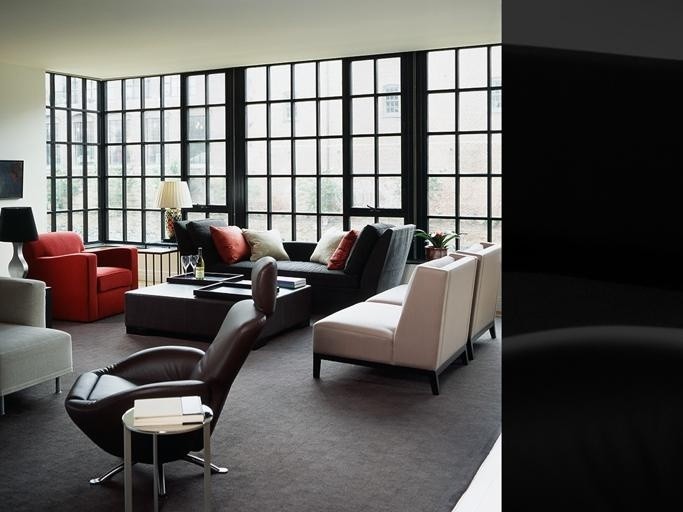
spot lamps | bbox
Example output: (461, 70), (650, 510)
(0, 207), (39, 279)
(159, 179), (193, 244)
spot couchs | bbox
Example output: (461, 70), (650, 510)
(173, 218), (416, 312)
(66, 257), (276, 484)
(456, 241), (501, 359)
(21, 231), (138, 323)
(0, 277), (71, 416)
(313, 253), (478, 396)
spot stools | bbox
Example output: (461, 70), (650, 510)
(121, 403), (213, 512)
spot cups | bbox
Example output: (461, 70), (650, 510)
(181, 256), (190, 276)
(190, 255), (199, 275)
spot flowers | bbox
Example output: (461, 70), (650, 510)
(414, 229), (468, 247)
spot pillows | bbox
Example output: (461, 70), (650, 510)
(308, 227), (347, 264)
(209, 225), (250, 263)
(344, 224), (395, 274)
(327, 229), (358, 269)
(187, 219), (225, 261)
(242, 227), (293, 261)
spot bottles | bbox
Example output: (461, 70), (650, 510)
(196, 248), (205, 282)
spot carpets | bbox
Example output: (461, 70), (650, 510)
(2, 312), (501, 512)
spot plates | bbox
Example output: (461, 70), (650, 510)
(193, 281), (279, 300)
(166, 271), (244, 285)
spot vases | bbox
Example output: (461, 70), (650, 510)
(425, 246), (447, 260)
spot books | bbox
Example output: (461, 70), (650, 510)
(132, 395), (205, 428)
(276, 276), (307, 289)
